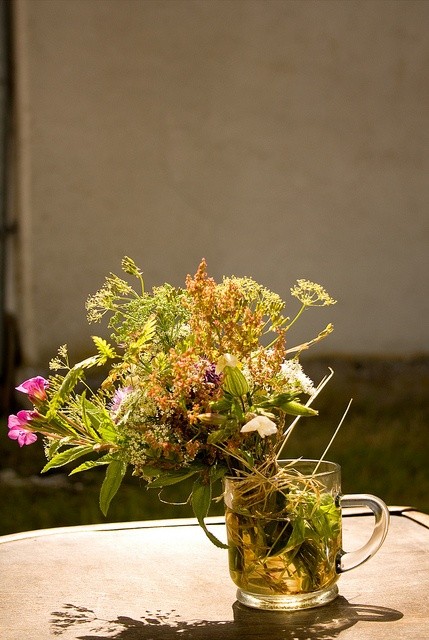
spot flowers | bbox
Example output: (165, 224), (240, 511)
(7, 250), (342, 593)
